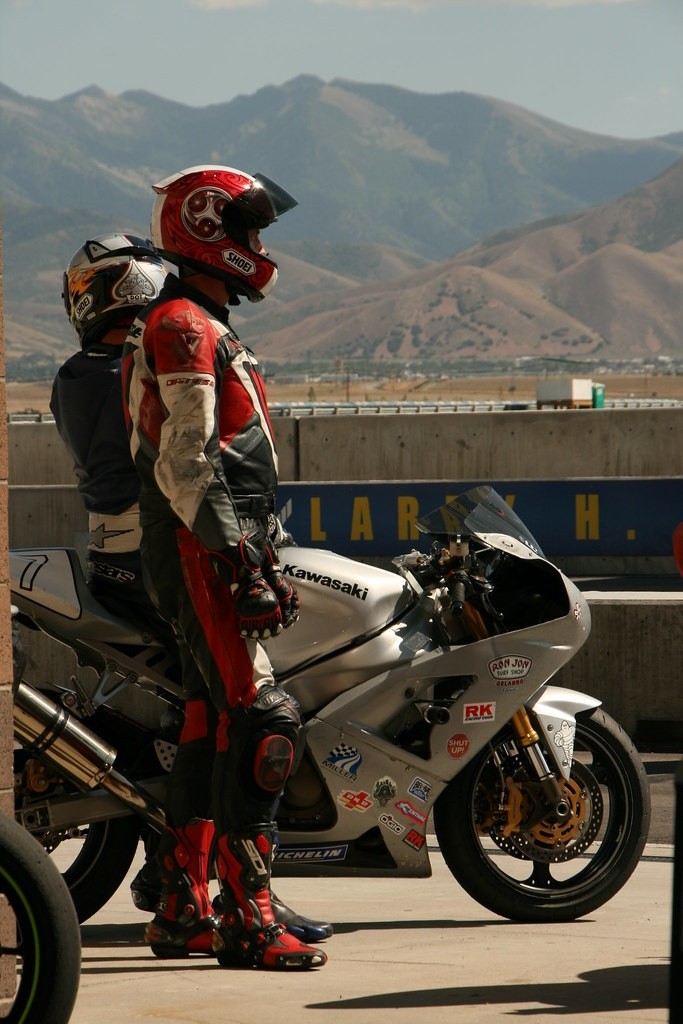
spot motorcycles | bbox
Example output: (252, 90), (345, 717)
(9, 483), (654, 944)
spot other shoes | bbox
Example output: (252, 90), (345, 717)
(267, 889), (334, 941)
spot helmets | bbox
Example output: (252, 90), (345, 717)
(62, 230), (169, 348)
(151, 164), (280, 301)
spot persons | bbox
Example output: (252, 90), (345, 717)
(116, 163), (326, 973)
(50, 235), (337, 940)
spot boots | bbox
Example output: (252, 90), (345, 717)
(147, 817), (220, 958)
(213, 825), (327, 970)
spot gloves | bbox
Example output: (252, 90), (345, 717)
(265, 572), (301, 631)
(229, 570), (284, 643)
